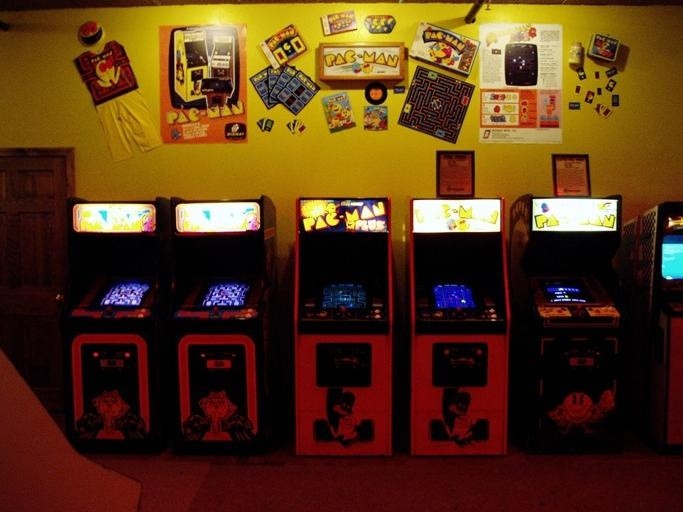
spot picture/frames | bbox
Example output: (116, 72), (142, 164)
(318, 41), (405, 84)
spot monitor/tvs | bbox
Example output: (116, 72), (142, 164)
(199, 280), (250, 307)
(537, 278), (594, 304)
(320, 282), (369, 309)
(661, 234), (683, 280)
(431, 282), (477, 310)
(98, 279), (151, 307)
